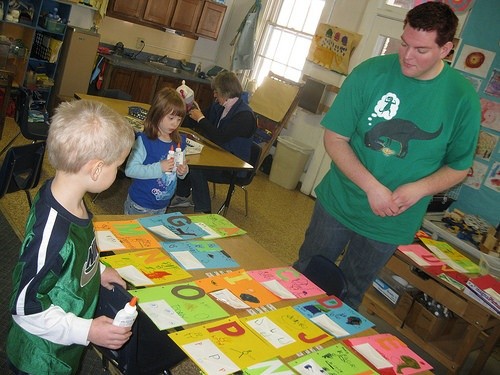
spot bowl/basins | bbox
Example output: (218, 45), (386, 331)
(478, 253), (500, 279)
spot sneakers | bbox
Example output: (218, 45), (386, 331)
(167, 194), (191, 208)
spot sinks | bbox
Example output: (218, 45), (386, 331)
(157, 55), (168, 64)
(144, 61), (192, 76)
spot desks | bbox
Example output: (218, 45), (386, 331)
(74, 93), (253, 176)
(361, 241), (500, 375)
(89, 214), (455, 375)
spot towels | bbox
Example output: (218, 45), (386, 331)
(207, 65), (225, 79)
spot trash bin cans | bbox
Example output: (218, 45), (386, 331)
(269, 136), (315, 190)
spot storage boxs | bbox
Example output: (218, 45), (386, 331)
(371, 266), (419, 305)
(394, 293), (467, 341)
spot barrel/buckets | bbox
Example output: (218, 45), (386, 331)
(268, 135), (315, 190)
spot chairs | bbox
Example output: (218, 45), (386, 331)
(201, 142), (262, 218)
(15, 88), (50, 144)
(0, 140), (46, 208)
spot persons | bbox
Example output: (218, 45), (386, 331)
(291, 1), (487, 311)
(3, 99), (135, 375)
(125, 87), (188, 215)
(168, 71), (257, 214)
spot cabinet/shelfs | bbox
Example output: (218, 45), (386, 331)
(152, 75), (199, 110)
(0, 0), (72, 125)
(89, 55), (157, 105)
(106, 0), (228, 42)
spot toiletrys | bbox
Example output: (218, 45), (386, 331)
(196, 62), (202, 74)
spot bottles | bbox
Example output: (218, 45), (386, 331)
(111, 296), (139, 330)
(165, 142), (183, 174)
(418, 297), (444, 317)
